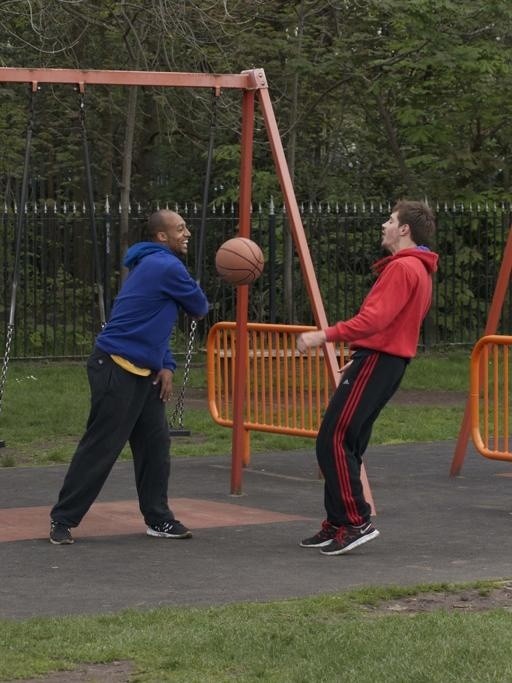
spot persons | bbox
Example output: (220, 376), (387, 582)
(49, 209), (211, 545)
(295, 197), (438, 556)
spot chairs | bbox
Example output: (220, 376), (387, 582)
(299, 519), (380, 555)
(146, 518), (194, 538)
(49, 519), (75, 545)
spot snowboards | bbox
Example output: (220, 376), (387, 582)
(215, 238), (264, 284)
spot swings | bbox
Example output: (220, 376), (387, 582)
(76, 79), (216, 434)
(0, 83), (36, 448)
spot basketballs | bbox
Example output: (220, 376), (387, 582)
(215, 238), (264, 284)
